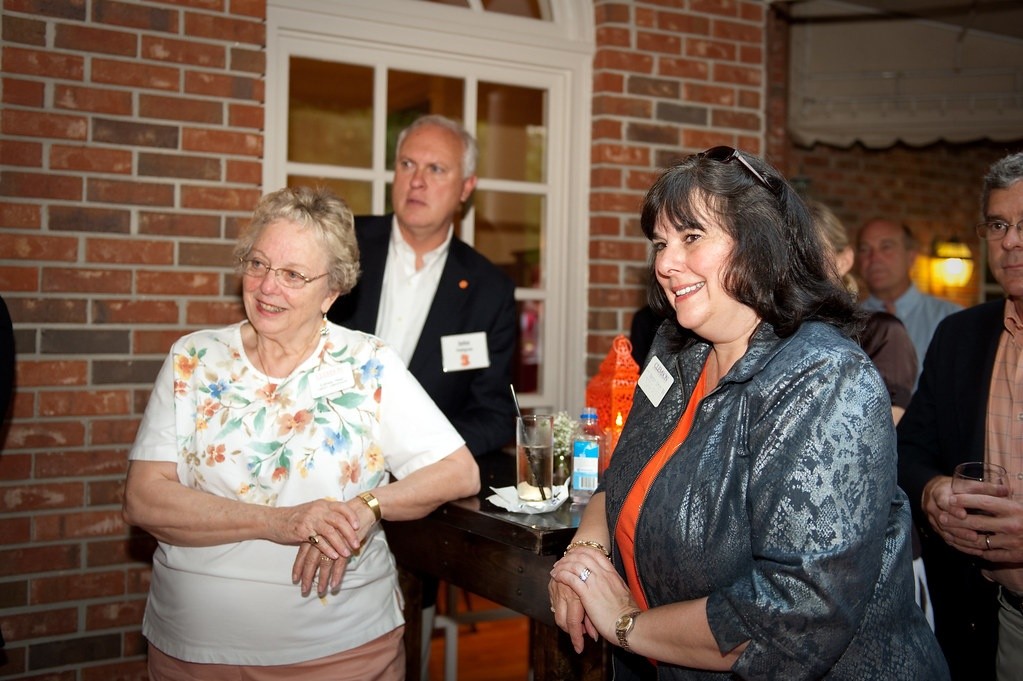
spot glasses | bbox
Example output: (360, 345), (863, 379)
(975, 221), (1023, 241)
(678, 146), (778, 195)
(239, 258), (329, 289)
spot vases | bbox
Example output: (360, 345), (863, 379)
(553, 456), (570, 485)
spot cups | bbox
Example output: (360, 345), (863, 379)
(516, 415), (554, 500)
(952, 462), (1011, 536)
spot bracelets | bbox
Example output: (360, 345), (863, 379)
(564, 540), (611, 561)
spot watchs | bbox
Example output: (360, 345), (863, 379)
(356, 492), (381, 521)
(616, 611), (642, 652)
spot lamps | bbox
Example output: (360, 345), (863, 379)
(937, 236), (973, 287)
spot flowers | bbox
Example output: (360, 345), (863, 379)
(547, 411), (579, 456)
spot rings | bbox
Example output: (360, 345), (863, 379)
(551, 607), (555, 612)
(308, 534), (318, 543)
(319, 553), (332, 561)
(986, 534), (992, 551)
(580, 568), (592, 581)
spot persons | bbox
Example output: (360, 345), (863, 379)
(329, 115), (522, 681)
(549, 146), (954, 680)
(808, 203), (919, 428)
(858, 221), (963, 392)
(121, 183), (481, 681)
(896, 154), (1023, 681)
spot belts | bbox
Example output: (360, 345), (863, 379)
(1000, 586), (1023, 614)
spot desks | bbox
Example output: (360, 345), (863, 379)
(405, 490), (623, 681)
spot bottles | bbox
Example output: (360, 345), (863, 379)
(570, 407), (605, 504)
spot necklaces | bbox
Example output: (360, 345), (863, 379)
(257, 322), (321, 404)
